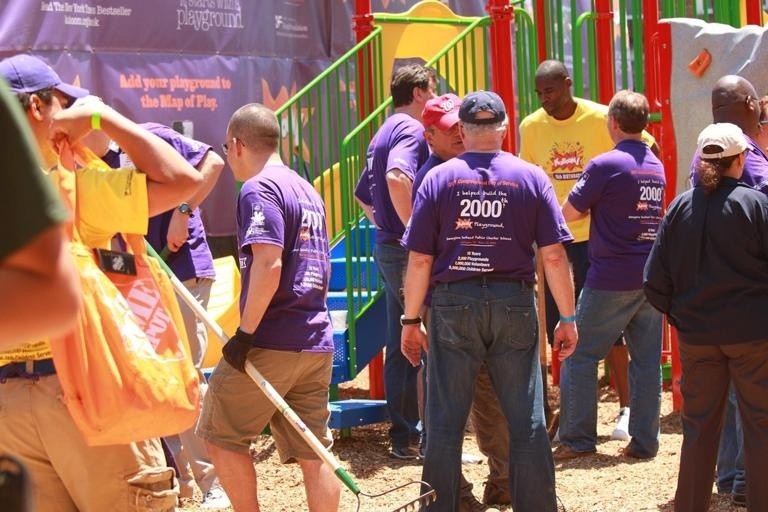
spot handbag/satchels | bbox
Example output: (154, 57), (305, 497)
(38, 133), (202, 447)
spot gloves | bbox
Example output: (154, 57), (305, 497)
(221, 325), (259, 374)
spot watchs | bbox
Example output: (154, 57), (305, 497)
(399, 314), (422, 325)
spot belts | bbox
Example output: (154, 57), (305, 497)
(0, 358), (54, 379)
(433, 274), (536, 287)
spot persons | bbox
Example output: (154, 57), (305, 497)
(517, 59), (659, 442)
(410, 94), (513, 512)
(690, 73), (768, 506)
(752, 96), (768, 160)
(356, 62), (437, 461)
(552, 89), (666, 462)
(195, 101), (341, 512)
(400, 92), (580, 512)
(643, 122), (768, 512)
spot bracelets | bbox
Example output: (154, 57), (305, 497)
(559, 314), (576, 321)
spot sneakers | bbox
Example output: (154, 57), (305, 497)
(716, 485), (747, 505)
(612, 405), (632, 440)
(391, 445), (421, 457)
(459, 494), (499, 512)
(483, 482), (512, 503)
(200, 487), (231, 509)
(621, 440), (648, 458)
(552, 445), (598, 460)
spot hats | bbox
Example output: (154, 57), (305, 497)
(0, 54), (90, 103)
(420, 93), (463, 132)
(458, 89), (507, 126)
(697, 123), (754, 160)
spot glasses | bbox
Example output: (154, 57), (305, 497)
(221, 137), (245, 155)
